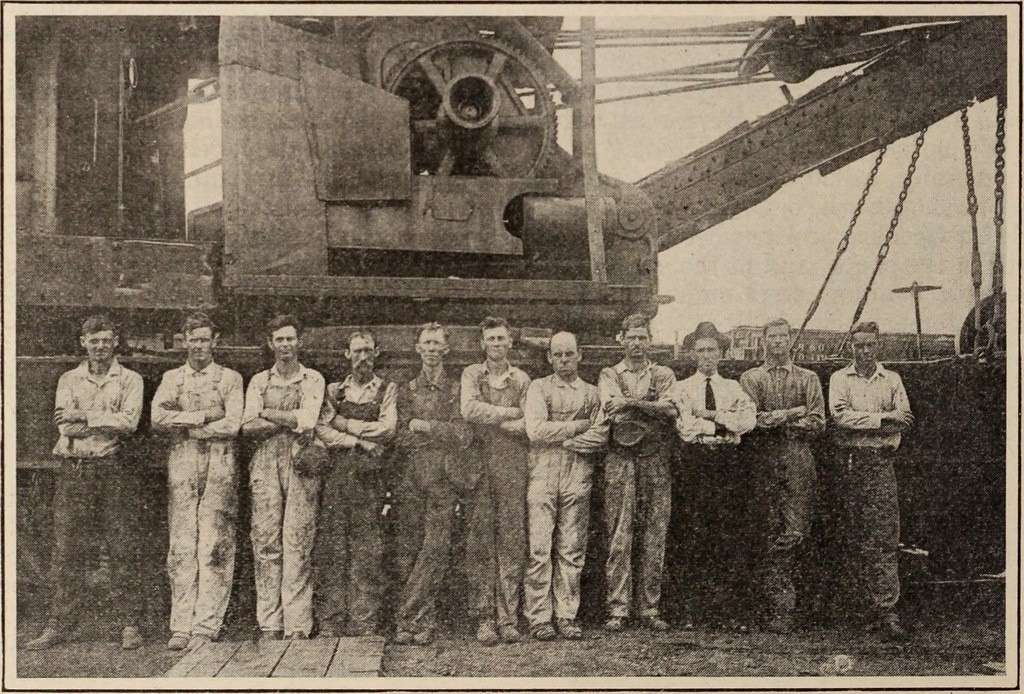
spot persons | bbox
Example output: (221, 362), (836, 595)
(523, 331), (610, 639)
(150, 312), (244, 650)
(828, 321), (915, 630)
(672, 321), (756, 634)
(461, 314), (531, 645)
(240, 315), (325, 642)
(598, 315), (680, 631)
(739, 317), (826, 634)
(395, 321), (472, 643)
(315, 331), (397, 637)
(28, 314), (144, 650)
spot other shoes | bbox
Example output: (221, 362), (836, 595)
(601, 615), (630, 632)
(477, 623), (499, 645)
(557, 619), (584, 641)
(875, 621), (910, 641)
(767, 615), (797, 634)
(257, 630), (282, 641)
(394, 627), (413, 644)
(167, 631), (189, 649)
(26, 626), (78, 651)
(414, 627), (435, 645)
(187, 634), (214, 651)
(500, 624), (523, 643)
(531, 623), (557, 641)
(121, 626), (144, 650)
(641, 617), (672, 632)
(284, 631), (310, 641)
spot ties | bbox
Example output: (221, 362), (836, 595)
(706, 378), (716, 411)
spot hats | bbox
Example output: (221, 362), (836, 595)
(683, 322), (731, 351)
(609, 407), (664, 456)
